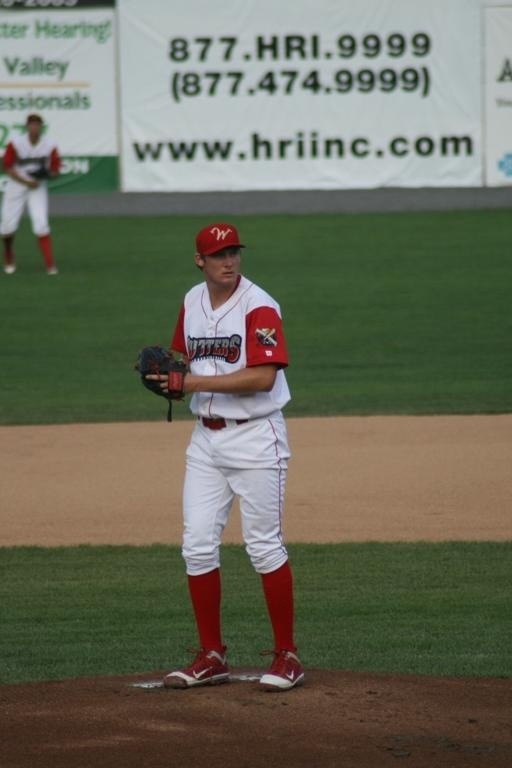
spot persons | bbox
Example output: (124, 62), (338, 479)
(132, 220), (308, 692)
(2, 112), (62, 277)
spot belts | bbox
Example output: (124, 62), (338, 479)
(199, 415), (249, 431)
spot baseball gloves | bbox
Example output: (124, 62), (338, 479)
(28, 167), (52, 179)
(134, 346), (190, 422)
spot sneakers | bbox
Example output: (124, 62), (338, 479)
(4, 264), (56, 275)
(259, 647), (305, 692)
(162, 645), (232, 686)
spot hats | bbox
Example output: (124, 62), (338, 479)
(196, 221), (244, 257)
(28, 112), (42, 121)
